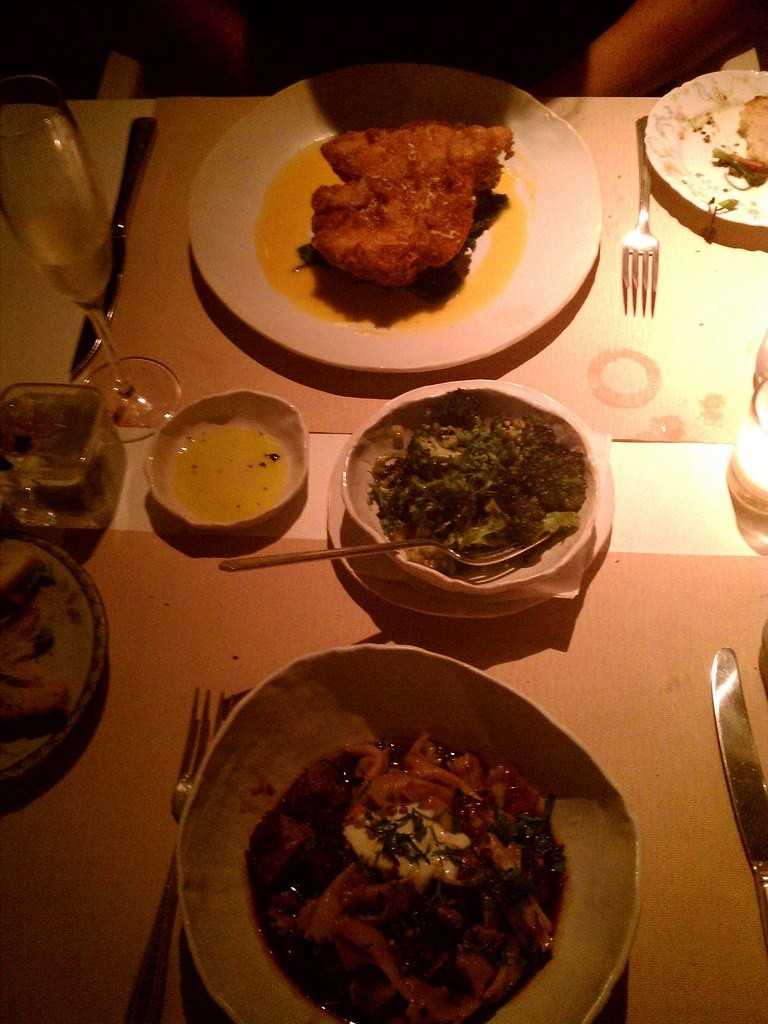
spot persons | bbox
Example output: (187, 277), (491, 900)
(166, 1), (768, 97)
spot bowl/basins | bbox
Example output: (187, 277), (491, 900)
(340, 386), (596, 594)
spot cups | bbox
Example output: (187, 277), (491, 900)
(724, 378), (768, 516)
(0, 381), (129, 531)
(751, 328), (768, 390)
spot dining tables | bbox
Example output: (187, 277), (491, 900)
(0, 95), (768, 1024)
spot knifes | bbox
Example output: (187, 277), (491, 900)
(69, 113), (159, 377)
(708, 646), (768, 950)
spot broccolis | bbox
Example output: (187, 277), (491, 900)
(392, 390), (587, 551)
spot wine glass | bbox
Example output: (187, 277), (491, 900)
(0, 73), (182, 443)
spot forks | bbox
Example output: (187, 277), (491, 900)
(620, 115), (661, 317)
(211, 526), (552, 573)
(124, 684), (238, 1024)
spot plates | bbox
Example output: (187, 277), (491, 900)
(644, 67), (768, 229)
(0, 533), (108, 783)
(146, 389), (311, 536)
(186, 59), (609, 375)
(176, 642), (642, 1024)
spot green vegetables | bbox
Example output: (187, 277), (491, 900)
(376, 791), (565, 890)
